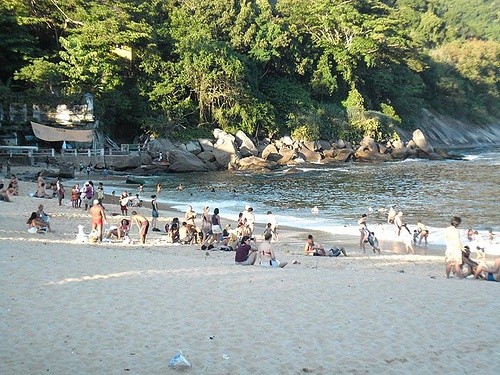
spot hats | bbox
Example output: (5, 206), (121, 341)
(92, 199), (100, 204)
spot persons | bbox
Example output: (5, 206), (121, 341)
(359, 222), (383, 255)
(34, 203), (55, 233)
(304, 234), (321, 256)
(0, 129), (164, 216)
(131, 210), (149, 244)
(366, 202), (495, 248)
(151, 194), (161, 232)
(256, 232), (301, 269)
(104, 218), (130, 241)
(25, 212), (48, 235)
(357, 213), (368, 253)
(368, 231), (381, 255)
(165, 182), (279, 253)
(400, 225), (415, 255)
(444, 215), (467, 280)
(234, 235), (258, 266)
(469, 257), (500, 282)
(267, 130), (396, 160)
(310, 204), (320, 212)
(452, 246), (487, 281)
(312, 244), (349, 258)
(89, 199), (107, 242)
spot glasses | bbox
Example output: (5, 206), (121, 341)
(245, 240), (251, 242)
